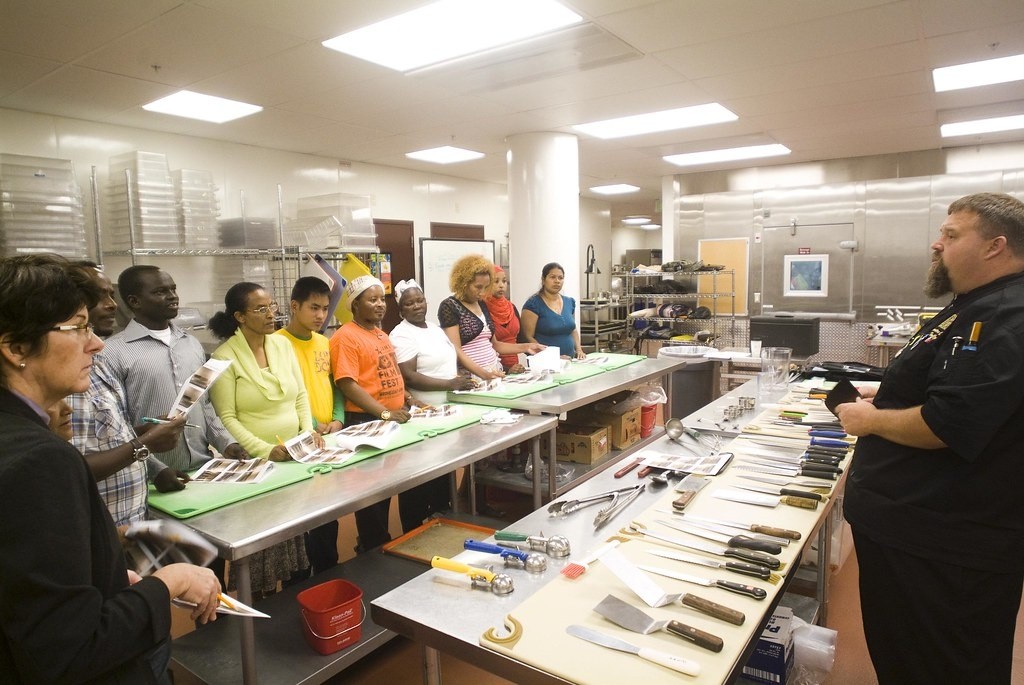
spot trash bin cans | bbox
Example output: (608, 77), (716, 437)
(657, 346), (722, 420)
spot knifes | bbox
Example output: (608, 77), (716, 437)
(567, 387), (852, 675)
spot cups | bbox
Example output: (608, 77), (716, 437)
(760, 346), (793, 392)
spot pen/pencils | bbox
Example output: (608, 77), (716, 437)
(149, 478), (193, 485)
(410, 405), (431, 415)
(486, 363), (497, 372)
(141, 417), (201, 429)
(453, 373), (461, 378)
(276, 434), (286, 446)
(968, 321), (982, 348)
(521, 364), (526, 368)
(217, 595), (238, 611)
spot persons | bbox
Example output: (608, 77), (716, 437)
(0, 250), (221, 685)
(45, 264), (249, 638)
(520, 263), (586, 362)
(835, 195), (1024, 685)
(210, 275), (438, 605)
(389, 255), (542, 521)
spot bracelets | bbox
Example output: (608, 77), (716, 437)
(406, 395), (413, 405)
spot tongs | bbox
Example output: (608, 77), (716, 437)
(547, 484), (645, 528)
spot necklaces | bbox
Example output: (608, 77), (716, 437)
(352, 320), (381, 340)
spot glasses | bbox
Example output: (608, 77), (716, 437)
(46, 322), (95, 340)
(244, 304), (277, 315)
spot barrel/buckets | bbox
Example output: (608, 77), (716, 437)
(297, 578), (367, 654)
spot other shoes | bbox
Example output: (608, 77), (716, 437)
(457, 492), (505, 518)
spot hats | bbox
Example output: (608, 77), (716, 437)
(346, 274), (385, 310)
(394, 278), (423, 307)
(493, 265), (504, 274)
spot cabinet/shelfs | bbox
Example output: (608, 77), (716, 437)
(99, 245), (380, 330)
(447, 353), (687, 512)
(370, 431), (854, 685)
(581, 302), (628, 354)
(145, 401), (558, 685)
(682, 370), (882, 620)
(624, 261), (735, 357)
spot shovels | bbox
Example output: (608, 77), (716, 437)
(595, 544), (745, 626)
(566, 625), (701, 678)
(671, 517), (791, 547)
(655, 518), (782, 555)
(637, 560), (765, 602)
(592, 594), (724, 654)
(646, 548), (770, 581)
(685, 512), (802, 541)
(636, 526), (781, 571)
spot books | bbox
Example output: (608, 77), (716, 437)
(172, 592), (270, 618)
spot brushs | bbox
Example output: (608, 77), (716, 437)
(561, 539), (621, 580)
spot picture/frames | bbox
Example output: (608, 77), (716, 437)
(783, 254), (828, 297)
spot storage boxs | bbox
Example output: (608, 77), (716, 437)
(569, 397), (641, 450)
(741, 604), (796, 685)
(538, 423), (612, 465)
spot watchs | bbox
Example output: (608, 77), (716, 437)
(380, 409), (391, 421)
(129, 438), (150, 461)
